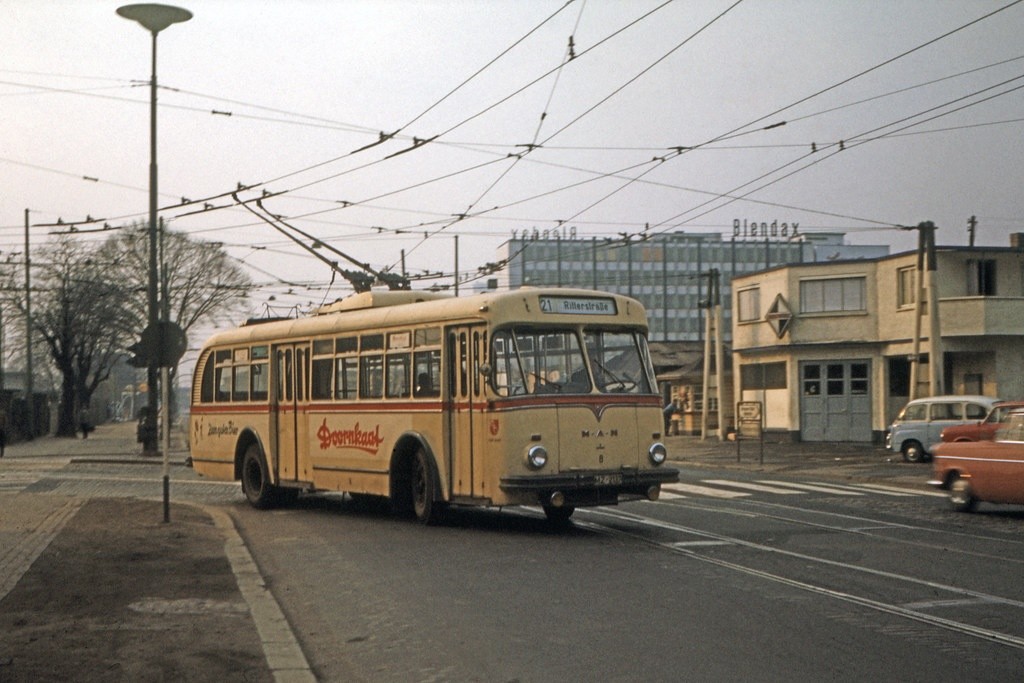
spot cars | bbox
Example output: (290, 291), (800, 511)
(924, 406), (1024, 511)
(940, 401), (1024, 441)
(886, 396), (1005, 462)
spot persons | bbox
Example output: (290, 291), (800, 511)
(0, 402), (8, 457)
(663, 399), (680, 436)
(136, 418), (146, 443)
(418, 373), (438, 394)
(805, 382), (820, 395)
(79, 406), (90, 439)
(572, 348), (607, 385)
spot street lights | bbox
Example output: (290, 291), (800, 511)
(112, 1), (195, 522)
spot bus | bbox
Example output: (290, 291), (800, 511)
(189, 285), (679, 529)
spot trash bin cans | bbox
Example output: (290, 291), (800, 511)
(670, 419), (679, 434)
